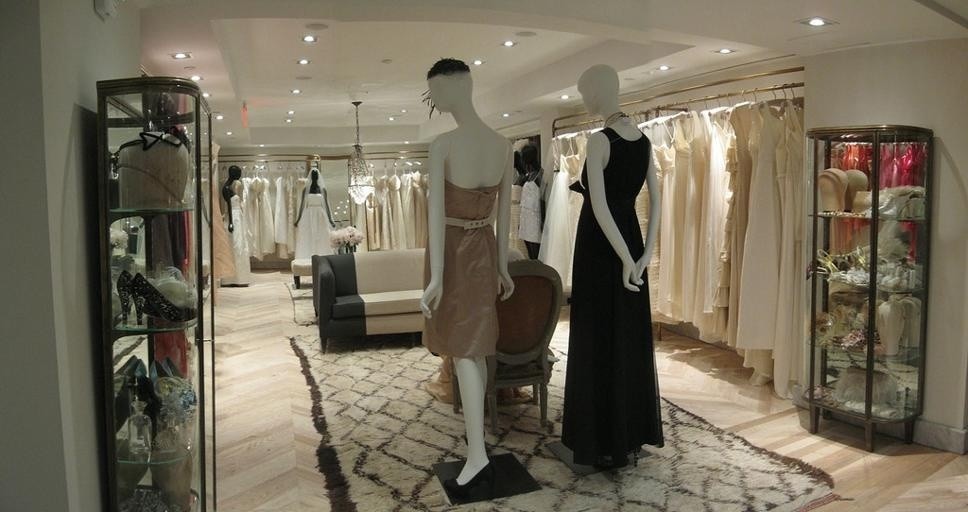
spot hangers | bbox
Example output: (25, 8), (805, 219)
(544, 82), (804, 144)
(220, 160), (305, 178)
(363, 156), (424, 178)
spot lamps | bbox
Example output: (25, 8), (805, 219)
(344, 99), (375, 206)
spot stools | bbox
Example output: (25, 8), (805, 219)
(290, 256), (314, 291)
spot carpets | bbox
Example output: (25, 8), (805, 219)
(287, 331), (855, 512)
(284, 276), (314, 325)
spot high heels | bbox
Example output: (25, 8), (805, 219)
(117, 270), (197, 325)
(878, 191), (926, 219)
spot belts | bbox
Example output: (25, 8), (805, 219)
(445, 217), (490, 230)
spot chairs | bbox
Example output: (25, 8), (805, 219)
(451, 259), (563, 436)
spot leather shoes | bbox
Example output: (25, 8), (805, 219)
(444, 463), (495, 491)
(150, 357), (197, 404)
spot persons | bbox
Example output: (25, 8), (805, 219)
(863, 295), (922, 356)
(818, 167), (849, 212)
(845, 169), (869, 212)
(508, 151), (529, 260)
(561, 64), (664, 472)
(221, 165), (251, 288)
(519, 144), (542, 259)
(418, 58), (514, 491)
(292, 168), (336, 259)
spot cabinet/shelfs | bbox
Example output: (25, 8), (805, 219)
(95, 76), (219, 512)
(806, 123), (938, 449)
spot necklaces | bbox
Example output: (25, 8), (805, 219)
(604, 112), (630, 129)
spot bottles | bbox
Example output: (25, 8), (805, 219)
(127, 402), (152, 456)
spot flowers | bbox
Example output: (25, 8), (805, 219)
(327, 224), (363, 252)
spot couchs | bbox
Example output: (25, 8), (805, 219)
(310, 248), (526, 358)
